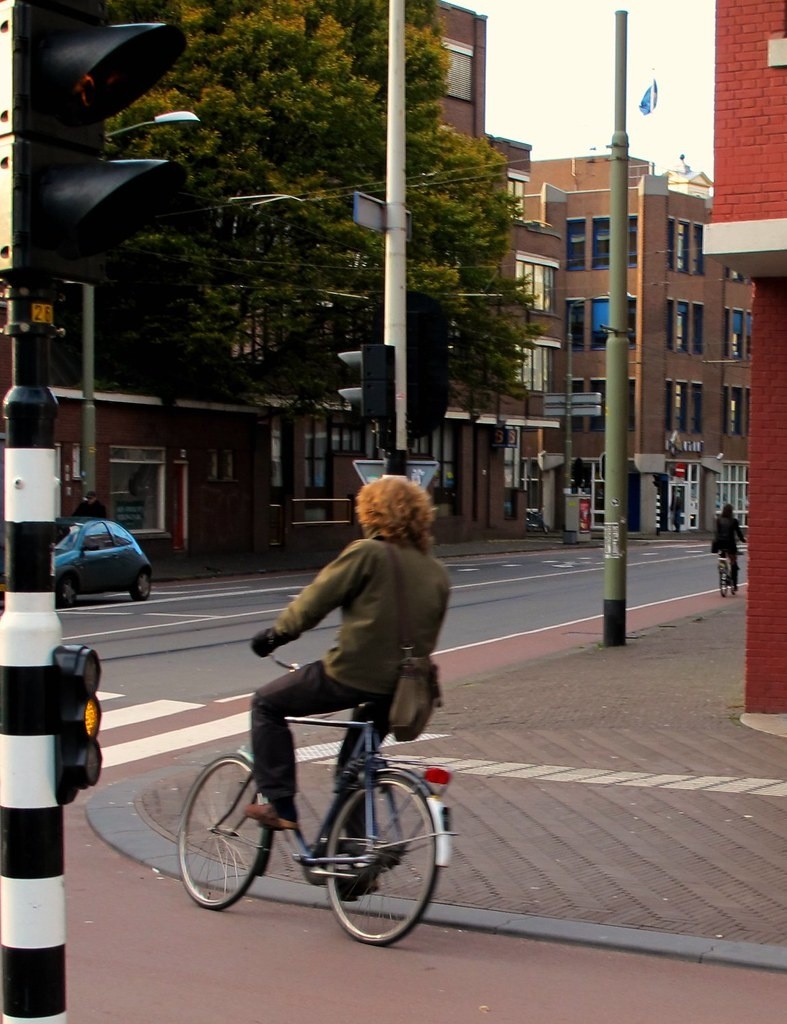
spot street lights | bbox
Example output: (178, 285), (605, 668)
(563, 291), (638, 491)
(78, 112), (201, 512)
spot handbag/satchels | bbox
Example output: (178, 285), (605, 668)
(389, 654), (441, 742)
(711, 540), (719, 554)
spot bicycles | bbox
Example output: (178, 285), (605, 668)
(176, 646), (462, 947)
(711, 539), (746, 598)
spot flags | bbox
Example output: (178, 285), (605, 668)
(640, 79), (657, 115)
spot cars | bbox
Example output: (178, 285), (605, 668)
(0, 517), (152, 604)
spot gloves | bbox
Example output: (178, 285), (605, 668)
(250, 625), (288, 658)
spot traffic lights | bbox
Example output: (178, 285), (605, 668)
(1, 2), (192, 290)
(338, 341), (394, 426)
(52, 647), (106, 810)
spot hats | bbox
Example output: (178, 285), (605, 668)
(85, 490), (96, 497)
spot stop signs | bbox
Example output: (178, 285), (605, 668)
(676, 463), (687, 477)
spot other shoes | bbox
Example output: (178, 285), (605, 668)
(245, 802), (299, 830)
(337, 876), (379, 898)
(732, 582), (738, 591)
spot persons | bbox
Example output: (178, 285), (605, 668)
(670, 488), (682, 533)
(711, 504), (747, 591)
(243, 476), (451, 901)
(72, 491), (106, 518)
